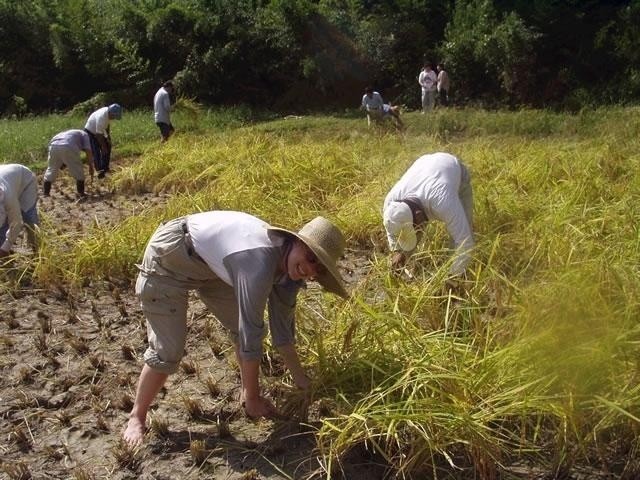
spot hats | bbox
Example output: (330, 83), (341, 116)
(107, 103), (122, 121)
(383, 200), (418, 253)
(266, 216), (352, 302)
(389, 106), (402, 115)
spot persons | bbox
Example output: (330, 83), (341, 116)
(153, 81), (175, 141)
(43, 129), (94, 196)
(383, 152), (475, 302)
(84, 103), (121, 178)
(419, 61), (437, 114)
(361, 88), (385, 130)
(383, 104), (404, 130)
(122, 210), (351, 448)
(0, 163), (41, 255)
(437, 64), (450, 107)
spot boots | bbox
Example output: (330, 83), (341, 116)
(43, 180), (49, 201)
(78, 181), (84, 198)
